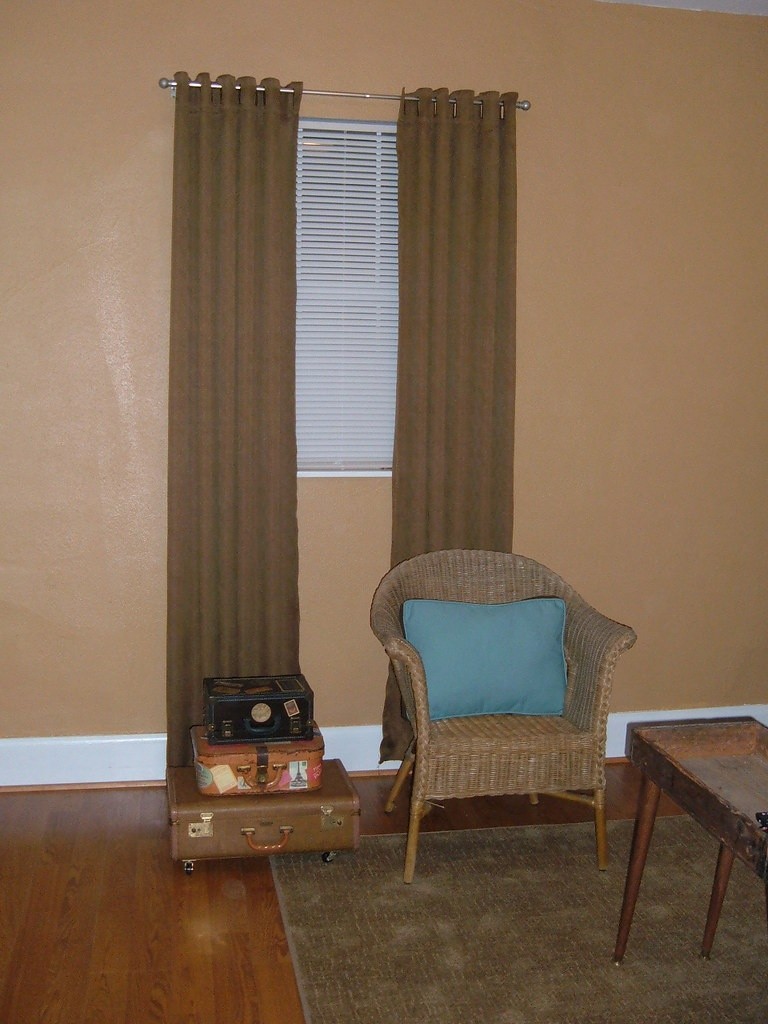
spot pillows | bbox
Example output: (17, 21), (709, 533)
(402, 598), (569, 720)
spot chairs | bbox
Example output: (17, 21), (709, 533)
(369, 548), (637, 884)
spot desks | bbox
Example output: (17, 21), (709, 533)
(613, 716), (768, 969)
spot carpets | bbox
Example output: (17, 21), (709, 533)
(268, 812), (768, 1024)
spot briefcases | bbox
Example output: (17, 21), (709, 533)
(202, 673), (314, 745)
(166, 759), (361, 875)
(190, 720), (325, 797)
